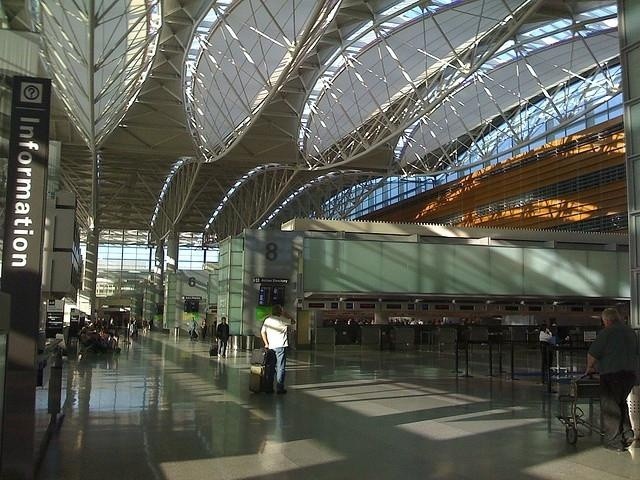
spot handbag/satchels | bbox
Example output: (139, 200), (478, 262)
(250, 348), (278, 365)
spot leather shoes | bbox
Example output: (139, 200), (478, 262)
(277, 389), (287, 394)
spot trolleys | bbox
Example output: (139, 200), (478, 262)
(558, 369), (607, 444)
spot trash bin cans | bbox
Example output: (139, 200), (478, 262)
(175, 327), (180, 336)
(229, 334), (255, 350)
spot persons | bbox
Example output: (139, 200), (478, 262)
(80, 316), (230, 358)
(550, 326), (570, 366)
(261, 304), (295, 395)
(538, 325), (553, 373)
(585, 306), (640, 452)
(390, 318), (424, 326)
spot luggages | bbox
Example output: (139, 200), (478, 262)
(248, 363), (275, 394)
(209, 338), (218, 356)
